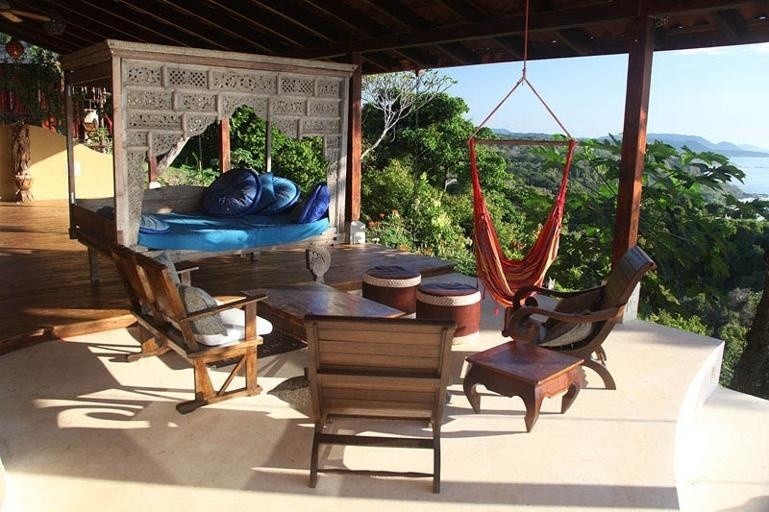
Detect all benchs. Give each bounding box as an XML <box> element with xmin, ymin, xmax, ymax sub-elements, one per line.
<box><xmin>104</xmin><ymin>237</ymin><xmax>267</xmax><ymax>413</ymax></box>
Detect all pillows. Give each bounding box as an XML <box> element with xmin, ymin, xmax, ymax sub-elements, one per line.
<box><xmin>200</xmin><ymin>167</ymin><xmax>327</xmax><ymax>224</ymax></box>
<box><xmin>151</xmin><ymin>252</ymin><xmax>225</xmax><ymax>337</ymax></box>
<box><xmin>542</xmin><ymin>288</ymin><xmax>600</xmax><ymax>346</ymax></box>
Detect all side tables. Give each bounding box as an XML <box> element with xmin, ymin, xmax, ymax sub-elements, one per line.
<box><xmin>462</xmin><ymin>340</ymin><xmax>587</xmax><ymax>430</ymax></box>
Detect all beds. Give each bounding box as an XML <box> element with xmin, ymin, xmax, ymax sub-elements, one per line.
<box><xmin>60</xmin><ymin>182</ymin><xmax>362</xmax><ymax>288</ymax></box>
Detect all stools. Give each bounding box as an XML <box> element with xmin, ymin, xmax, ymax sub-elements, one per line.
<box><xmin>363</xmin><ymin>265</ymin><xmax>483</xmax><ymax>335</ymax></box>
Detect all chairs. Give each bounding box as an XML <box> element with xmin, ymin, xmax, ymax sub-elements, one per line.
<box><xmin>502</xmin><ymin>247</ymin><xmax>654</xmax><ymax>389</ymax></box>
<box><xmin>305</xmin><ymin>315</ymin><xmax>456</xmax><ymax>494</ymax></box>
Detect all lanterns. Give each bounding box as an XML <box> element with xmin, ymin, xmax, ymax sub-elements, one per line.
<box><xmin>5</xmin><ymin>37</ymin><xmax>25</xmax><ymax>60</ymax></box>
